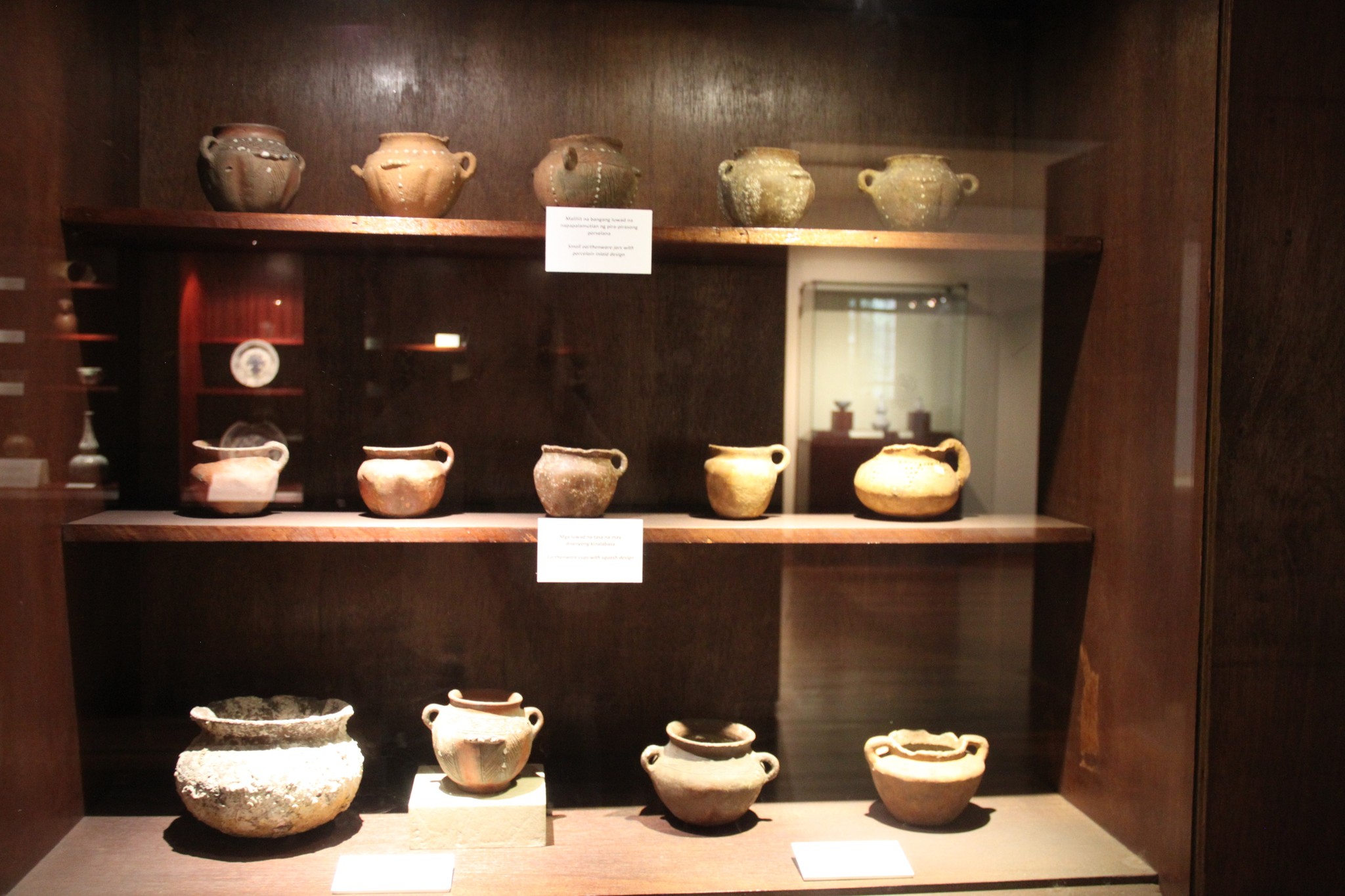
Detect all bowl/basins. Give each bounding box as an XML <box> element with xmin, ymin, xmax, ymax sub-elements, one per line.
<box><xmin>76</xmin><ymin>366</ymin><xmax>107</xmax><ymax>385</ymax></box>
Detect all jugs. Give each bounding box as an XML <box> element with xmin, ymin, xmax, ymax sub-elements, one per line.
<box><xmin>355</xmin><ymin>442</ymin><xmax>455</xmax><ymax>519</ymax></box>
<box><xmin>533</xmin><ymin>443</ymin><xmax>628</xmax><ymax>518</ymax></box>
<box><xmin>852</xmin><ymin>436</ymin><xmax>972</xmax><ymax>520</ymax></box>
<box><xmin>702</xmin><ymin>443</ymin><xmax>793</xmax><ymax>519</ymax></box>
<box><xmin>183</xmin><ymin>436</ymin><xmax>290</xmax><ymax>517</ymax></box>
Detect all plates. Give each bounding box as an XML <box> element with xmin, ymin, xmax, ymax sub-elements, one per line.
<box><xmin>231</xmin><ymin>338</ymin><xmax>280</xmax><ymax>388</ymax></box>
<box><xmin>217</xmin><ymin>420</ymin><xmax>288</xmax><ymax>461</ymax></box>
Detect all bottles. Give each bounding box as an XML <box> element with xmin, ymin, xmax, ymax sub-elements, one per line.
<box><xmin>640</xmin><ymin>717</ymin><xmax>780</xmax><ymax>828</ymax></box>
<box><xmin>350</xmin><ymin>132</ymin><xmax>478</xmax><ymax>218</ymax></box>
<box><xmin>717</xmin><ymin>145</ymin><xmax>816</xmax><ymax>228</ymax></box>
<box><xmin>421</xmin><ymin>686</ymin><xmax>544</xmax><ymax>794</ymax></box>
<box><xmin>863</xmin><ymin>728</ymin><xmax>990</xmax><ymax>827</ymax></box>
<box><xmin>173</xmin><ymin>694</ymin><xmax>366</xmax><ymax>839</ymax></box>
<box><xmin>65</xmin><ymin>410</ymin><xmax>110</xmax><ymax>484</ymax></box>
<box><xmin>53</xmin><ymin>297</ymin><xmax>77</xmax><ymax>334</ymax></box>
<box><xmin>858</xmin><ymin>152</ymin><xmax>981</xmax><ymax>232</ymax></box>
<box><xmin>531</xmin><ymin>133</ymin><xmax>642</xmax><ymax>211</ymax></box>
<box><xmin>196</xmin><ymin>122</ymin><xmax>306</xmax><ymax>213</ymax></box>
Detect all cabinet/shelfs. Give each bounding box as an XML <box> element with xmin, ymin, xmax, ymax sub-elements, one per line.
<box><xmin>0</xmin><ymin>204</ymin><xmax>1158</xmax><ymax>896</ymax></box>
